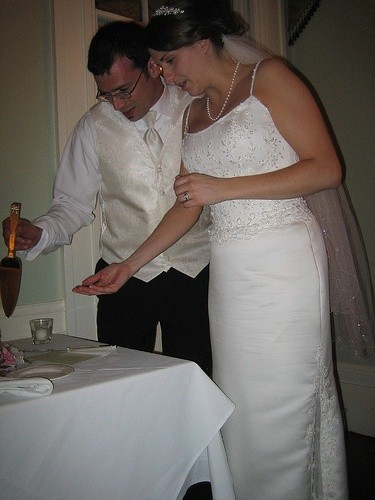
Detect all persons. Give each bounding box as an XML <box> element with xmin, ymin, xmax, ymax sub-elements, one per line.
<box><xmin>72</xmin><ymin>0</ymin><xmax>375</xmax><ymax>500</ymax></box>
<box><xmin>2</xmin><ymin>21</ymin><xmax>212</xmax><ymax>382</ymax></box>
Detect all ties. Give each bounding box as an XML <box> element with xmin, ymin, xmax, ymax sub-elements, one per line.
<box><xmin>142</xmin><ymin>109</ymin><xmax>164</xmax><ymax>162</ymax></box>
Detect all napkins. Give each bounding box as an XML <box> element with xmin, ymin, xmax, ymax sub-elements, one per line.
<box><xmin>0</xmin><ymin>377</ymin><xmax>54</xmax><ymax>396</ymax></box>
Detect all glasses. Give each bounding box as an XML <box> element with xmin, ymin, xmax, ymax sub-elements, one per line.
<box><xmin>95</xmin><ymin>69</ymin><xmax>146</xmax><ymax>102</ymax></box>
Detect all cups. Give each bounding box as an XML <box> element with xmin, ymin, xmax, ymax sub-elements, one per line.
<box><xmin>29</xmin><ymin>318</ymin><xmax>53</xmax><ymax>344</ymax></box>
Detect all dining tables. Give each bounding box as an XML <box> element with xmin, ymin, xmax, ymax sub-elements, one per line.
<box><xmin>0</xmin><ymin>334</ymin><xmax>235</xmax><ymax>500</ymax></box>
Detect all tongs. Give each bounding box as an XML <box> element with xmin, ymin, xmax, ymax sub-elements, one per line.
<box><xmin>67</xmin><ymin>343</ymin><xmax>117</xmax><ymax>353</ymax></box>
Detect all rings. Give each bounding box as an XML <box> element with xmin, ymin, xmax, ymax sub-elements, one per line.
<box><xmin>184</xmin><ymin>192</ymin><xmax>188</xmax><ymax>201</ymax></box>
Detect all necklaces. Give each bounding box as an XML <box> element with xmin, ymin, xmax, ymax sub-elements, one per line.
<box><xmin>207</xmin><ymin>62</ymin><xmax>241</xmax><ymax>120</ymax></box>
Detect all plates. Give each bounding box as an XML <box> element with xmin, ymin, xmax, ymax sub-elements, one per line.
<box><xmin>14</xmin><ymin>364</ymin><xmax>75</xmax><ymax>382</ymax></box>
